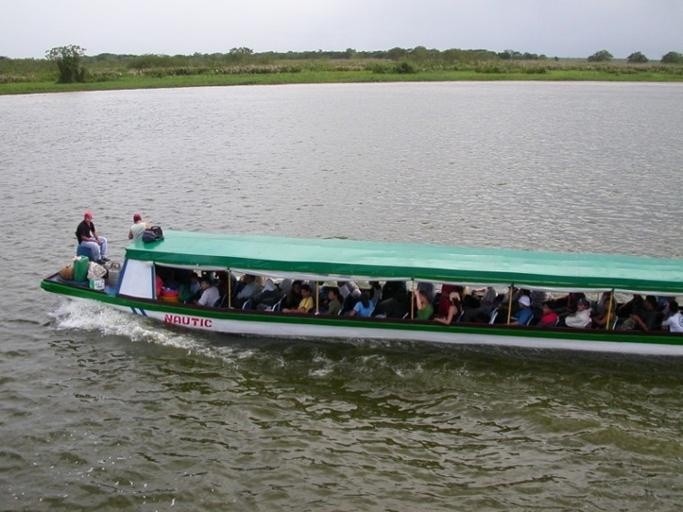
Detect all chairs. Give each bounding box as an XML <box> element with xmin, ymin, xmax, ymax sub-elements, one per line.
<box><xmin>213</xmin><ymin>286</ymin><xmax>619</xmax><ymax>331</ymax></box>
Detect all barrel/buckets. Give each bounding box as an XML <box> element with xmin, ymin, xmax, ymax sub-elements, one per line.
<box><xmin>74</xmin><ymin>255</ymin><xmax>88</xmax><ymax>281</ymax></box>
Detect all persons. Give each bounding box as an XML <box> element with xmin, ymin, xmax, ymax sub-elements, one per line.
<box><xmin>129</xmin><ymin>214</ymin><xmax>146</xmax><ymax>239</ymax></box>
<box><xmin>156</xmin><ymin>267</ymin><xmax>236</xmax><ymax>309</ymax></box>
<box><xmin>76</xmin><ymin>212</ymin><xmax>111</xmax><ymax>263</ymax></box>
<box><xmin>496</xmin><ymin>285</ymin><xmax>682</xmax><ymax>334</ymax></box>
<box><xmin>236</xmin><ymin>274</ymin><xmax>360</xmax><ymax>317</ymax></box>
<box><xmin>347</xmin><ymin>280</ymin><xmax>498</xmax><ymax>326</ymax></box>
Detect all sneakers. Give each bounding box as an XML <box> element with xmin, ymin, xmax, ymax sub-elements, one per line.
<box><xmin>97</xmin><ymin>259</ymin><xmax>105</xmax><ymax>264</ymax></box>
<box><xmin>101</xmin><ymin>257</ymin><xmax>110</xmax><ymax>261</ymax></box>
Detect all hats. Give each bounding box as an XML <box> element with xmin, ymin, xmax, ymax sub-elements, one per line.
<box><xmin>85</xmin><ymin>213</ymin><xmax>93</xmax><ymax>219</ymax></box>
<box><xmin>351</xmin><ymin>290</ymin><xmax>361</xmax><ymax>298</ymax></box>
<box><xmin>261</xmin><ymin>279</ymin><xmax>277</xmax><ymax>292</ymax></box>
<box><xmin>450</xmin><ymin>292</ymin><xmax>461</xmax><ymax>301</ymax></box>
<box><xmin>518</xmin><ymin>296</ymin><xmax>531</xmax><ymax>306</ymax></box>
<box><xmin>578</xmin><ymin>299</ymin><xmax>587</xmax><ymax>306</ymax></box>
<box><xmin>134</xmin><ymin>214</ymin><xmax>140</xmax><ymax>221</ymax></box>
<box><xmin>359</xmin><ymin>281</ymin><xmax>372</xmax><ymax>290</ymax></box>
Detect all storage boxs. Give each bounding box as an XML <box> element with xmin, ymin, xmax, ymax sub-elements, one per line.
<box><xmin>89</xmin><ymin>279</ymin><xmax>106</xmax><ymax>290</ymax></box>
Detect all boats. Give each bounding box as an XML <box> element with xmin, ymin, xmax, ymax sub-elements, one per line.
<box><xmin>39</xmin><ymin>230</ymin><xmax>683</xmax><ymax>357</ymax></box>
